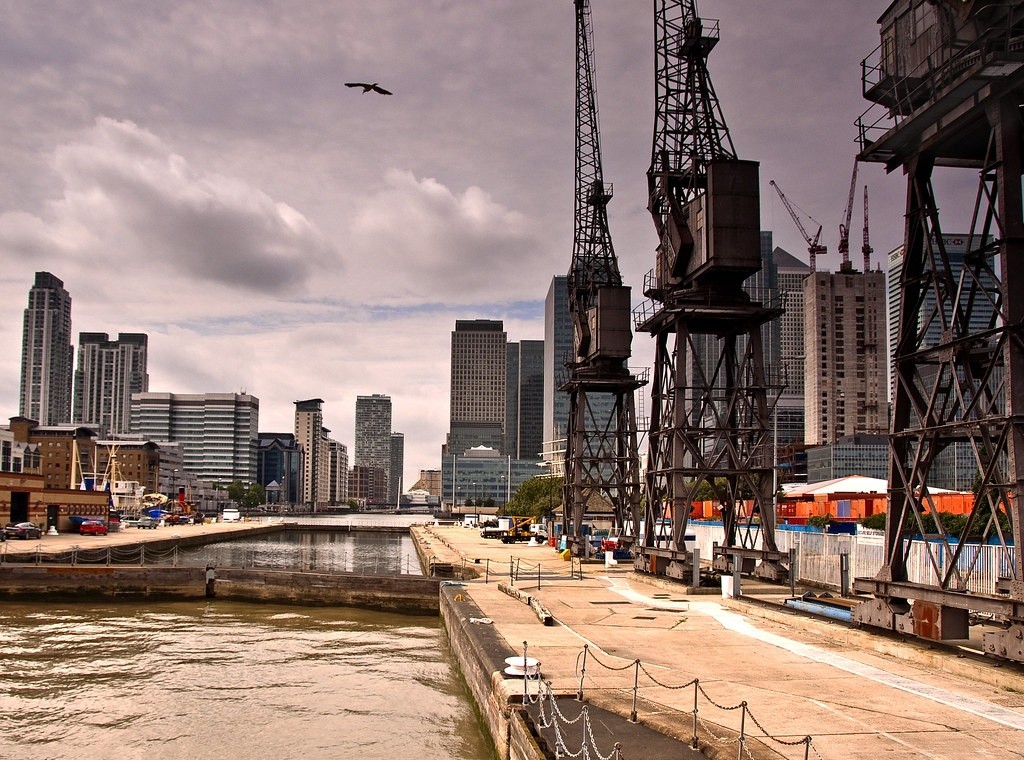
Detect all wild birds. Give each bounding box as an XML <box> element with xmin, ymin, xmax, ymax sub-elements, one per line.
<box><xmin>344</xmin><ymin>81</ymin><xmax>394</xmax><ymax>96</ymax></box>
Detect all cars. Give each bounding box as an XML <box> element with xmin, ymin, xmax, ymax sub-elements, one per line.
<box><xmin>137</xmin><ymin>517</ymin><xmax>157</xmax><ymax>530</ymax></box>
<box><xmin>4</xmin><ymin>522</ymin><xmax>43</xmax><ymax>540</ymax></box>
<box><xmin>121</xmin><ymin>516</ymin><xmax>139</xmax><ymax>527</ymax></box>
<box><xmin>80</xmin><ymin>520</ymin><xmax>108</xmax><ymax>536</ymax></box>
<box><xmin>0</xmin><ymin>523</ymin><xmax>7</xmax><ymax>542</ymax></box>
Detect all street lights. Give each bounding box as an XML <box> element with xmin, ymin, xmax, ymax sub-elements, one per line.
<box><xmin>247</xmin><ymin>481</ymin><xmax>252</xmax><ymax>517</ymax></box>
<box><xmin>473</xmin><ymin>483</ymin><xmax>477</xmax><ymax>528</ymax></box>
<box><xmin>216</xmin><ymin>478</ymin><xmax>222</xmax><ymax>517</ymax></box>
<box><xmin>457</xmin><ymin>487</ymin><xmax>461</xmax><ymax>522</ymax></box>
<box><xmin>173</xmin><ymin>468</ymin><xmax>178</xmax><ymax>516</ymax></box>
<box><xmin>501</xmin><ymin>475</ymin><xmax>506</xmax><ymax>516</ymax></box>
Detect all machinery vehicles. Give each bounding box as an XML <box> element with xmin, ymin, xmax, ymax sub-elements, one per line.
<box><xmin>497</xmin><ymin>513</ymin><xmax>549</xmax><ymax>545</ymax></box>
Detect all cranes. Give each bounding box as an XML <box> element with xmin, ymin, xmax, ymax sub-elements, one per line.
<box><xmin>549</xmin><ymin>0</ymin><xmax>799</xmax><ymax>598</ymax></box>
<box><xmin>769</xmin><ymin>178</ymin><xmax>830</xmax><ymax>273</ymax></box>
<box><xmin>835</xmin><ymin>154</ymin><xmax>875</xmax><ymax>274</ymax></box>
